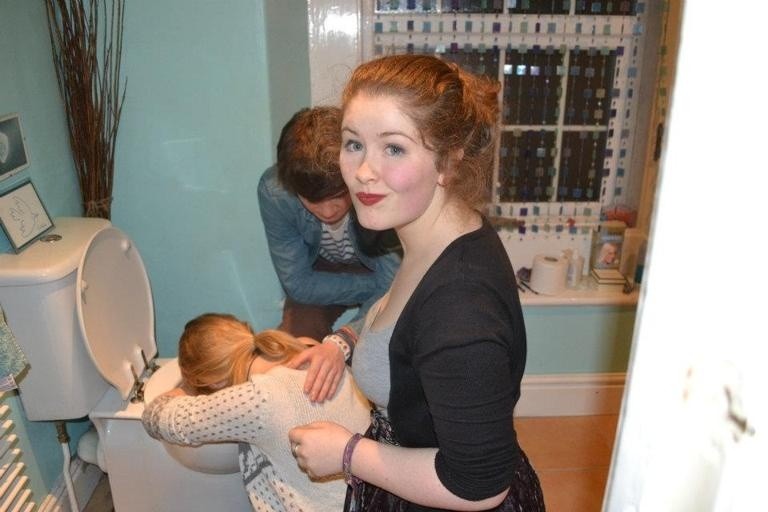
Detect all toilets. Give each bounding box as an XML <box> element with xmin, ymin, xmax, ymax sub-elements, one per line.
<box><xmin>0</xmin><ymin>218</ymin><xmax>253</xmax><ymax>512</ymax></box>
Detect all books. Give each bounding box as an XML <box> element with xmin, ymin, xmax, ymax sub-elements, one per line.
<box><xmin>590</xmin><ymin>268</ymin><xmax>627</xmax><ymax>285</ymax></box>
<box><xmin>589</xmin><ymin>220</ymin><xmax>627</xmax><ymax>270</ymax></box>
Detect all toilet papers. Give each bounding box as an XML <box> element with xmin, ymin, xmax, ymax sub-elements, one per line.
<box><xmin>530</xmin><ymin>254</ymin><xmax>567</xmax><ymax>296</ymax></box>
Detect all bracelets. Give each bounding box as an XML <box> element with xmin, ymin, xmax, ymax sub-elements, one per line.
<box><xmin>324</xmin><ymin>324</ymin><xmax>359</xmax><ymax>361</ymax></box>
<box><xmin>341</xmin><ymin>430</ymin><xmax>369</xmax><ymax>489</ymax></box>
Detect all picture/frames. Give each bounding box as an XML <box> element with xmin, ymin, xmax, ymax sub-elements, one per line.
<box><xmin>0</xmin><ymin>110</ymin><xmax>33</xmax><ymax>185</ymax></box>
<box><xmin>0</xmin><ymin>175</ymin><xmax>55</xmax><ymax>256</ymax></box>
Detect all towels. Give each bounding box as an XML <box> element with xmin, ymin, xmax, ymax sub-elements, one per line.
<box><xmin>1</xmin><ymin>305</ymin><xmax>29</xmax><ymax>402</ymax></box>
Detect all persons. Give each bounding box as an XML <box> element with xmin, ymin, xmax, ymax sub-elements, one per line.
<box><xmin>598</xmin><ymin>240</ymin><xmax>619</xmax><ymax>264</ymax></box>
<box><xmin>254</xmin><ymin>105</ymin><xmax>408</xmax><ymax>406</ymax></box>
<box><xmin>139</xmin><ymin>309</ymin><xmax>408</xmax><ymax>510</ymax></box>
<box><xmin>286</xmin><ymin>52</ymin><xmax>548</xmax><ymax>510</ymax></box>
